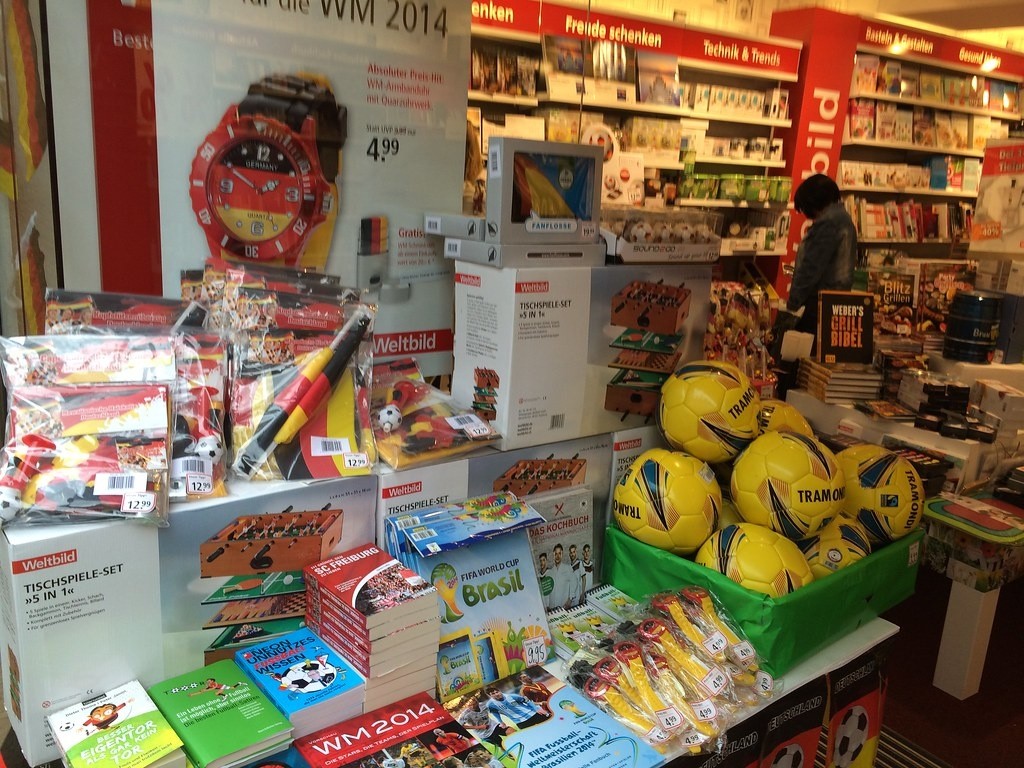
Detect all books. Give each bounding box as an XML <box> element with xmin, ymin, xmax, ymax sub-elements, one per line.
<box><xmin>46</xmin><ymin>483</ymin><xmax>667</xmax><ymax>768</ymax></box>
<box><xmin>795</xmin><ymin>51</ymin><xmax>1024</xmax><ymax>420</ymax></box>
<box><xmin>471</xmin><ymin>33</ymin><xmax>680</xmax><ymax>108</ymax></box>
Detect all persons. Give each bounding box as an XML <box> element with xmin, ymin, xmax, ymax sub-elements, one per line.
<box><xmin>568</xmin><ymin>544</ymin><xmax>587</xmax><ymax>593</ymax></box>
<box><xmin>398</xmin><ymin>741</ymin><xmax>426</xmax><ymax>768</ymax></box>
<box><xmin>580</xmin><ymin>544</ymin><xmax>593</xmax><ymax>591</ymax></box>
<box><xmin>517</xmin><ymin>671</ymin><xmax>552</xmax><ymax>705</ymax></box>
<box><xmin>483</xmin><ymin>685</ymin><xmax>546</xmax><ymax>730</ymax></box>
<box><xmin>377</xmin><ymin>756</ymin><xmax>411</xmax><ymax>768</ymax></box>
<box><xmin>786</xmin><ymin>173</ymin><xmax>858</xmax><ymax>357</ymax></box>
<box><xmin>441</xmin><ymin>756</ymin><xmax>466</xmax><ymax>768</ymax></box>
<box><xmin>475</xmin><ymin>748</ymin><xmax>506</xmax><ymax>768</ymax></box>
<box><xmin>547</xmin><ymin>545</ymin><xmax>580</xmax><ymax>608</ymax></box>
<box><xmin>464</xmin><ymin>752</ymin><xmax>482</xmax><ymax>768</ymax></box>
<box><xmin>457</xmin><ymin>696</ymin><xmax>517</xmax><ymax>749</ymax></box>
<box><xmin>536</xmin><ymin>553</ymin><xmax>553</xmax><ymax>612</ymax></box>
<box><xmin>432</xmin><ymin>728</ymin><xmax>472</xmax><ymax>755</ymax></box>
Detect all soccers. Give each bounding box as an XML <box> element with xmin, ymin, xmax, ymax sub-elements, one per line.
<box><xmin>612</xmin><ymin>358</ymin><xmax>925</xmax><ymax>606</ymax></box>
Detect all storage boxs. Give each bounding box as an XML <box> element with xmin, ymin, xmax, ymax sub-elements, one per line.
<box><xmin>0</xmin><ymin>73</ymin><xmax>791</xmax><ymax>767</ymax></box>
<box><xmin>599</xmin><ymin>521</ymin><xmax>927</xmax><ymax>680</ymax></box>
<box><xmin>838</xmin><ymin>51</ymin><xmax>1024</xmax><ymax>238</ymax></box>
<box><xmin>921</xmin><ymin>493</ymin><xmax>1024</xmax><ymax>594</ymax></box>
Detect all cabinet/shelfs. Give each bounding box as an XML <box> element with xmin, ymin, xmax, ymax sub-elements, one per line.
<box><xmin>463</xmin><ymin>23</ymin><xmax>804</xmax><ymax>256</ymax></box>
<box><xmin>835</xmin><ymin>45</ymin><xmax>1024</xmax><ymax>243</ymax></box>
